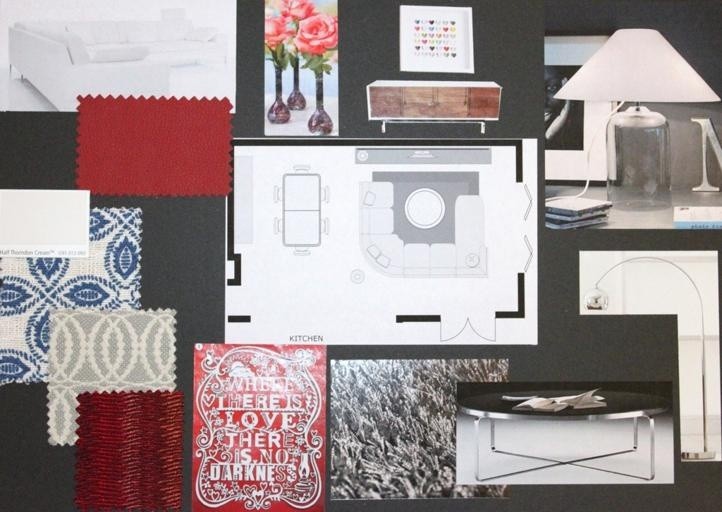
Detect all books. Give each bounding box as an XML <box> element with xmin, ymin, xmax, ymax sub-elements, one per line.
<box><xmin>672</xmin><ymin>206</ymin><xmax>722</xmax><ymax>231</ymax></box>
<box><xmin>544</xmin><ymin>197</ymin><xmax>614</xmax><ymax>231</ymax></box>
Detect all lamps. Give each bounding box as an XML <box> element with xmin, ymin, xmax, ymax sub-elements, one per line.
<box><xmin>552</xmin><ymin>27</ymin><xmax>722</xmax><ymax>213</ymax></box>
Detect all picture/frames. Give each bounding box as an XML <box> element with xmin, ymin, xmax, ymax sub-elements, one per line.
<box><xmin>543</xmin><ymin>31</ymin><xmax>624</xmax><ymax>188</ymax></box>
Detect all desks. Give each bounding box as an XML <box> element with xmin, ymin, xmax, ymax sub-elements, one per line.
<box><xmin>457</xmin><ymin>387</ymin><xmax>671</xmax><ymax>483</ymax></box>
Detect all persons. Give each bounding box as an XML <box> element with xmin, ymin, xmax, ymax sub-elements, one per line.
<box><xmin>543</xmin><ymin>66</ymin><xmax>572</xmax><ymax>150</ymax></box>
